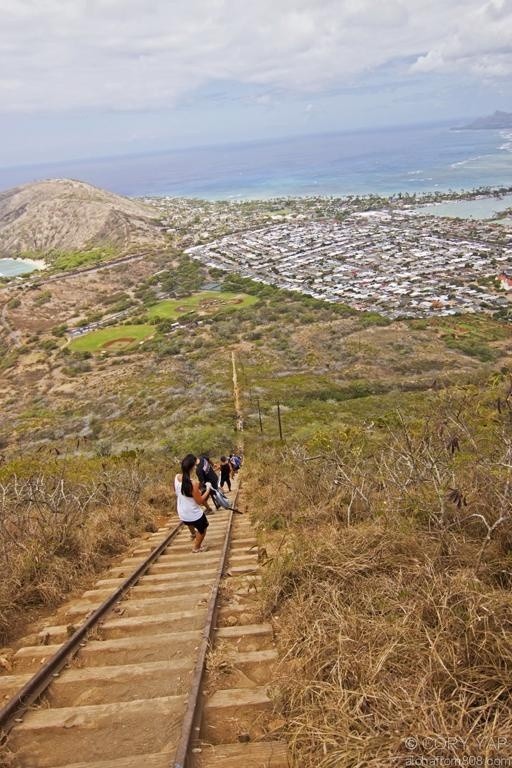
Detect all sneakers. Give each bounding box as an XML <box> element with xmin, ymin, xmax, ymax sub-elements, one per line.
<box><xmin>192</xmin><ymin>545</ymin><xmax>207</xmax><ymax>553</ymax></box>
<box><xmin>204</xmin><ymin>509</ymin><xmax>213</xmax><ymax>515</ymax></box>
<box><xmin>190</xmin><ymin>530</ymin><xmax>198</xmax><ymax>538</ymax></box>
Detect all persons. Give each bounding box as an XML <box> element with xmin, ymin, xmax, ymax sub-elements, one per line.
<box><xmin>175</xmin><ymin>451</ymin><xmax>241</xmax><ymax>553</ymax></box>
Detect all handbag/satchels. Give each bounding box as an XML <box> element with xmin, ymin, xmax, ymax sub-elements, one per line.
<box><xmin>215</xmin><ymin>491</ymin><xmax>230</xmax><ymax>508</ymax></box>
